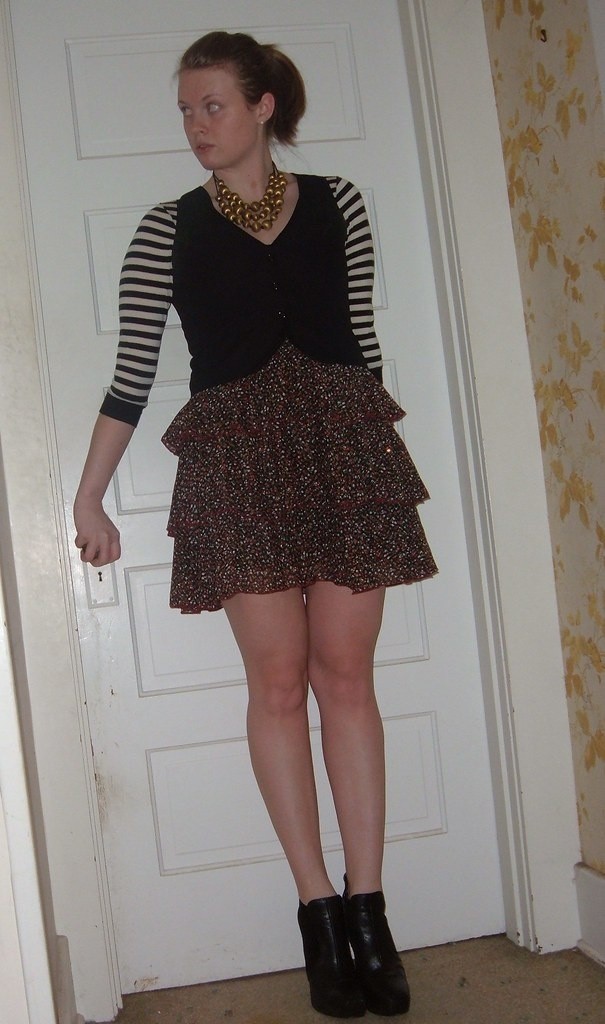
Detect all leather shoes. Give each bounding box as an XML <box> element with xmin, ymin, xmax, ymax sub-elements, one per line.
<box><xmin>298</xmin><ymin>895</ymin><xmax>367</xmax><ymax>1018</ymax></box>
<box><xmin>342</xmin><ymin>872</ymin><xmax>411</xmax><ymax>1016</ymax></box>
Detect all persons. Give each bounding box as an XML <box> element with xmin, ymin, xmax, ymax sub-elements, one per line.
<box><xmin>73</xmin><ymin>33</ymin><xmax>437</xmax><ymax>1020</ymax></box>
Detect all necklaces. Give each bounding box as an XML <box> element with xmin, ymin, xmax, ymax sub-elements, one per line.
<box><xmin>212</xmin><ymin>159</ymin><xmax>288</xmax><ymax>233</ymax></box>
